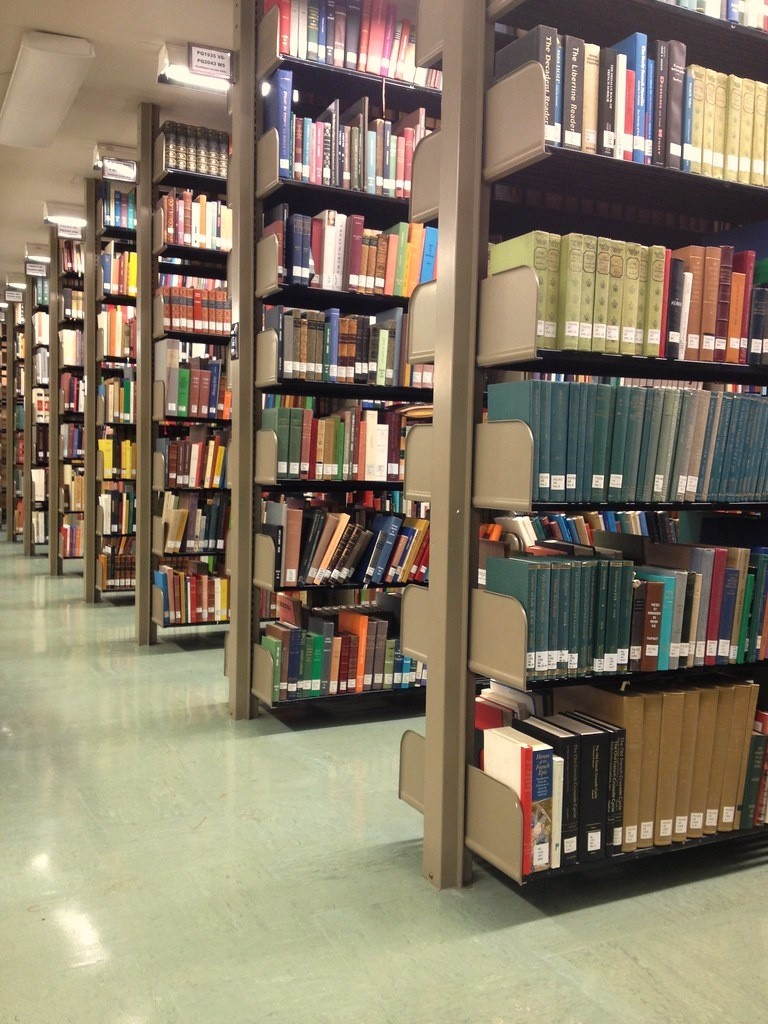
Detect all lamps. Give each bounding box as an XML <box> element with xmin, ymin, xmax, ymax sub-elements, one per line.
<box><xmin>0</xmin><ymin>295</ymin><xmax>9</xmax><ymax>308</ymax></box>
<box><xmin>92</xmin><ymin>144</ymin><xmax>135</xmax><ymax>169</ymax></box>
<box><xmin>5</xmin><ymin>272</ymin><xmax>27</xmax><ymax>289</ymax></box>
<box><xmin>24</xmin><ymin>243</ymin><xmax>51</xmax><ymax>262</ymax></box>
<box><xmin>156</xmin><ymin>43</ymin><xmax>230</xmax><ymax>94</ymax></box>
<box><xmin>41</xmin><ymin>202</ymin><xmax>88</xmax><ymax>226</ymax></box>
<box><xmin>0</xmin><ymin>30</ymin><xmax>97</xmax><ymax>151</ymax></box>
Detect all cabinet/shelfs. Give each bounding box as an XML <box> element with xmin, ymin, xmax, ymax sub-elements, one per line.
<box><xmin>0</xmin><ymin>304</ymin><xmax>10</xmax><ymax>533</ymax></box>
<box><xmin>49</xmin><ymin>225</ymin><xmax>88</xmax><ymax>575</ymax></box>
<box><xmin>5</xmin><ymin>287</ymin><xmax>25</xmax><ymax>544</ymax></box>
<box><xmin>229</xmin><ymin>0</ymin><xmax>427</xmax><ymax>728</ymax></box>
<box><xmin>135</xmin><ymin>100</ymin><xmax>233</xmax><ymax>646</ymax></box>
<box><xmin>21</xmin><ymin>259</ymin><xmax>51</xmax><ymax>561</ymax></box>
<box><xmin>400</xmin><ymin>0</ymin><xmax>765</xmax><ymax>896</ymax></box>
<box><xmin>81</xmin><ymin>174</ymin><xmax>140</xmax><ymax>604</ymax></box>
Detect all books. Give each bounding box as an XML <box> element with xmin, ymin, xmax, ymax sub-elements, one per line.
<box><xmin>0</xmin><ymin>1</ymin><xmax>768</xmax><ymax>884</ymax></box>
